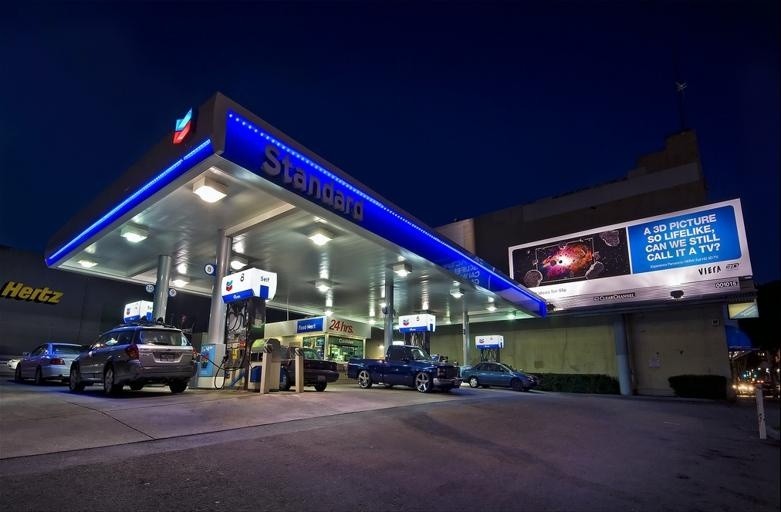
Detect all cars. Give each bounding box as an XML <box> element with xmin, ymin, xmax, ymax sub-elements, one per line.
<box><xmin>280</xmin><ymin>348</ymin><xmax>339</xmax><ymax>391</ymax></box>
<box><xmin>6</xmin><ymin>343</ymin><xmax>94</xmax><ymax>385</ymax></box>
<box><xmin>461</xmin><ymin>361</ymin><xmax>544</xmax><ymax>392</ymax></box>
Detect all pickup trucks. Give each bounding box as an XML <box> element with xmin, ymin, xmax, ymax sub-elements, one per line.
<box><xmin>347</xmin><ymin>345</ymin><xmax>463</xmax><ymax>392</ymax></box>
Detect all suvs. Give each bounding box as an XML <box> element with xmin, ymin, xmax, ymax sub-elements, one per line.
<box><xmin>69</xmin><ymin>319</ymin><xmax>197</xmax><ymax>393</ymax></box>
